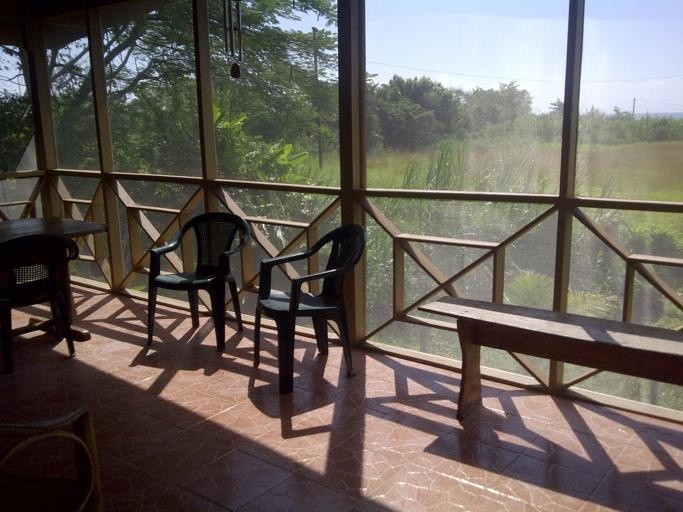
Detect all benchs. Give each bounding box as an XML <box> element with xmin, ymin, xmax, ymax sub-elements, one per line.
<box><xmin>417</xmin><ymin>296</ymin><xmax>683</xmax><ymax>420</ymax></box>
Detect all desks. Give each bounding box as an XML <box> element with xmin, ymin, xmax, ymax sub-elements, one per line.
<box><xmin>0</xmin><ymin>214</ymin><xmax>110</xmax><ymax>341</ymax></box>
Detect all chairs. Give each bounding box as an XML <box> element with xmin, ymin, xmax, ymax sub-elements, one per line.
<box><xmin>147</xmin><ymin>210</ymin><xmax>251</xmax><ymax>352</ymax></box>
<box><xmin>0</xmin><ymin>234</ymin><xmax>78</xmax><ymax>373</ymax></box>
<box><xmin>1</xmin><ymin>403</ymin><xmax>103</xmax><ymax>512</ymax></box>
<box><xmin>254</xmin><ymin>222</ymin><xmax>366</xmax><ymax>396</ymax></box>
